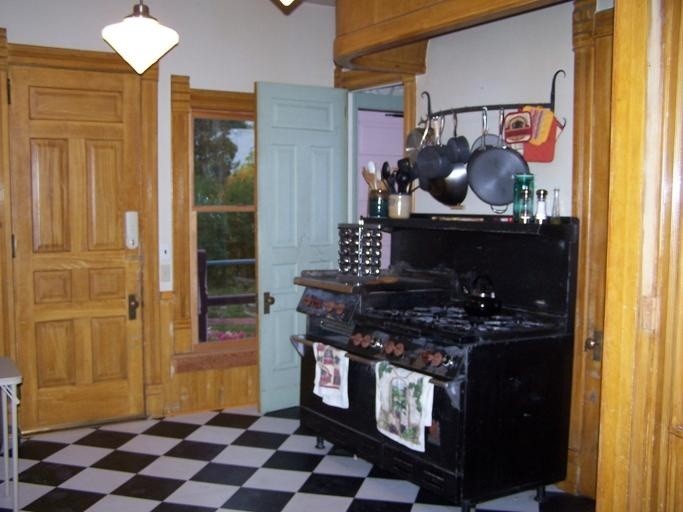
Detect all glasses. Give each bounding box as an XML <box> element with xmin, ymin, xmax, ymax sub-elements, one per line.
<box><xmin>101</xmin><ymin>0</ymin><xmax>180</xmax><ymax>75</ymax></box>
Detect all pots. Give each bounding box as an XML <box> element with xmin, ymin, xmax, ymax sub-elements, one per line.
<box><xmin>404</xmin><ymin>106</ymin><xmax>529</xmax><ymax>213</ymax></box>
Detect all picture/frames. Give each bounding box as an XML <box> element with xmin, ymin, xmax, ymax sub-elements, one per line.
<box><xmin>0</xmin><ymin>357</ymin><xmax>23</xmax><ymax>512</ymax></box>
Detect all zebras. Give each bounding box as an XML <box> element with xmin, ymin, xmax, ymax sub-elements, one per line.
<box><xmin>291</xmin><ymin>312</ymin><xmax>573</xmax><ymax>506</ymax></box>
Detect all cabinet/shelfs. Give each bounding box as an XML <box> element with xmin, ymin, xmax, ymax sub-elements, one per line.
<box><xmin>512</xmin><ymin>171</ymin><xmax>561</xmax><ymax>225</ymax></box>
<box><xmin>367</xmin><ymin>189</ymin><xmax>412</xmax><ymax>220</ymax></box>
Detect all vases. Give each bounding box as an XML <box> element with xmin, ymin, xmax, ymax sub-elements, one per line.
<box><xmin>206</xmin><ymin>330</ymin><xmax>247</xmax><ymax>341</ymax></box>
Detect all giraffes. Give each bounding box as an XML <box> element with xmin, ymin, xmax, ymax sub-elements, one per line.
<box><xmin>346</xmin><ymin>299</ymin><xmax>566</xmax><ymax>381</ymax></box>
